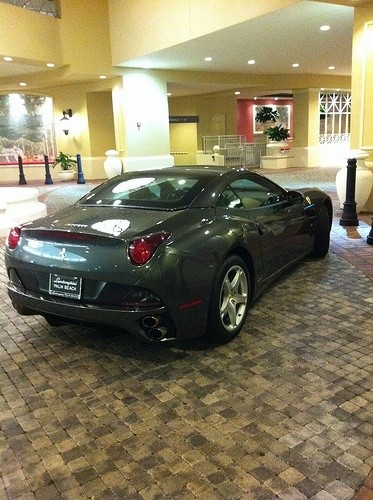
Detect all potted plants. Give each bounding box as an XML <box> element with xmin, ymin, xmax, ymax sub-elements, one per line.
<box><xmin>52</xmin><ymin>152</ymin><xmax>77</xmax><ymax>181</ymax></box>
<box><xmin>255</xmin><ymin>107</ymin><xmax>279</xmax><ymax>132</ymax></box>
<box><xmin>263</xmin><ymin>124</ymin><xmax>290</xmax><ymax>152</ymax></box>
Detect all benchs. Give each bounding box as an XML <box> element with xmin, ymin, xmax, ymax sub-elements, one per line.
<box><xmin>129</xmin><ymin>186</ymin><xmax>185</xmax><ymax>200</ymax></box>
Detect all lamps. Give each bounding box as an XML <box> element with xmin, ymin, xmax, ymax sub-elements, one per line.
<box><xmin>60</xmin><ymin>109</ymin><xmax>72</xmax><ymax>135</ymax></box>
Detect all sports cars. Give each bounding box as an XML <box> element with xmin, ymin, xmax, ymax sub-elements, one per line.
<box><xmin>3</xmin><ymin>164</ymin><xmax>334</xmax><ymax>347</ymax></box>
